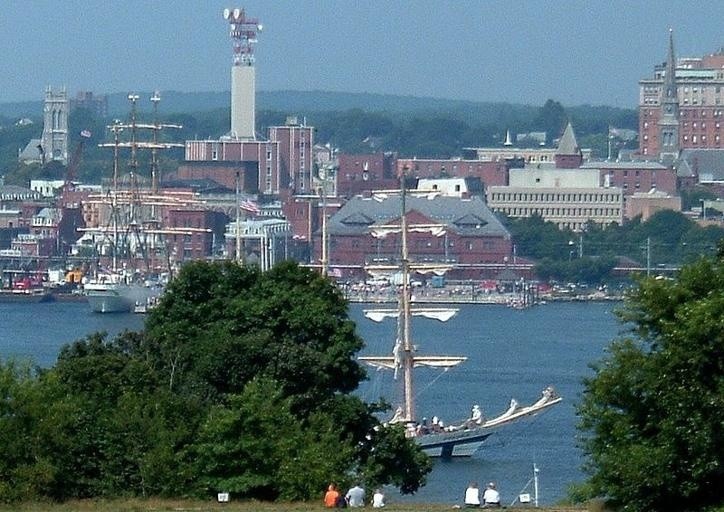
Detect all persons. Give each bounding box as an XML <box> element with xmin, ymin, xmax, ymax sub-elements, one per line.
<box><xmin>371</xmin><ymin>489</ymin><xmax>385</xmax><ymax>509</ymax></box>
<box><xmin>324</xmin><ymin>484</ymin><xmax>339</xmax><ymax>508</ymax></box>
<box><xmin>483</xmin><ymin>482</ymin><xmax>500</xmax><ymax>506</ymax></box>
<box><xmin>464</xmin><ymin>481</ymin><xmax>481</xmax><ymax>506</ymax></box>
<box><xmin>344</xmin><ymin>480</ymin><xmax>366</xmax><ymax>507</ymax></box>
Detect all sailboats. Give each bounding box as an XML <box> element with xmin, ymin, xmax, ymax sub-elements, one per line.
<box><xmin>336</xmin><ymin>167</ymin><xmax>564</xmax><ymax>459</ymax></box>
<box><xmin>82</xmin><ymin>92</ymin><xmax>211</xmax><ymax>314</ymax></box>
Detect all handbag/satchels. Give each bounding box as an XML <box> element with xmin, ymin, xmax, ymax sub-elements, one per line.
<box><xmin>336</xmin><ymin>497</ymin><xmax>346</xmax><ymax>508</ymax></box>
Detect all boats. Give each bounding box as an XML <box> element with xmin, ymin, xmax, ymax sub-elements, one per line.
<box><xmin>0</xmin><ymin>289</ymin><xmax>47</xmax><ymax>302</ymax></box>
<box><xmin>129</xmin><ymin>296</ymin><xmax>164</xmax><ymax>314</ymax></box>
